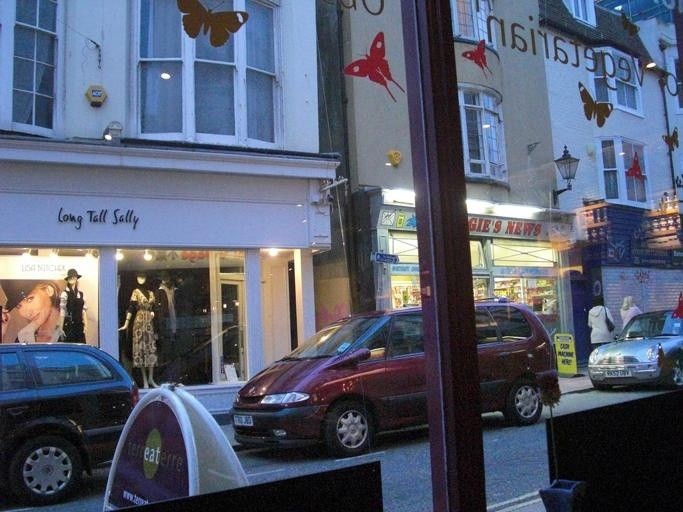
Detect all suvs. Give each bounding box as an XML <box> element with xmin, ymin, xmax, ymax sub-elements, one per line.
<box><xmin>0</xmin><ymin>338</ymin><xmax>144</xmax><ymax>509</ymax></box>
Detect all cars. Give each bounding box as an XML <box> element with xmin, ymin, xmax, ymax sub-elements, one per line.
<box><xmin>586</xmin><ymin>307</ymin><xmax>682</xmax><ymax>394</ymax></box>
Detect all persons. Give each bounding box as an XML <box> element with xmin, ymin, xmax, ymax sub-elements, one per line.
<box><xmin>0</xmin><ymin>280</ymin><xmax>59</xmax><ymax>344</ymax></box>
<box><xmin>154</xmin><ymin>270</ymin><xmax>187</xmax><ymax>387</ymax></box>
<box><xmin>118</xmin><ymin>272</ymin><xmax>161</xmax><ymax>389</ymax></box>
<box><xmin>54</xmin><ymin>268</ymin><xmax>87</xmax><ymax>344</ymax></box>
<box><xmin>587</xmin><ymin>296</ymin><xmax>613</xmax><ymax>347</ymax></box>
<box><xmin>618</xmin><ymin>295</ymin><xmax>642</xmax><ymax>330</ymax></box>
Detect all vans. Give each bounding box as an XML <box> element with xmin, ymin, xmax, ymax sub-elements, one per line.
<box><xmin>227</xmin><ymin>293</ymin><xmax>565</xmax><ymax>462</ymax></box>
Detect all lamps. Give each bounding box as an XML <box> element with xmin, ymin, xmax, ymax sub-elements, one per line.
<box><xmin>552</xmin><ymin>145</ymin><xmax>581</xmax><ymax>207</ymax></box>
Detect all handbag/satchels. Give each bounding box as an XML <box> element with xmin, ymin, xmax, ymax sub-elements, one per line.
<box><xmin>605</xmin><ymin>317</ymin><xmax>615</xmax><ymax>333</ymax></box>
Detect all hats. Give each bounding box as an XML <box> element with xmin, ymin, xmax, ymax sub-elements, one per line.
<box><xmin>64</xmin><ymin>269</ymin><xmax>82</xmax><ymax>281</ymax></box>
<box><xmin>0</xmin><ymin>280</ymin><xmax>43</xmax><ymax>312</ymax></box>
